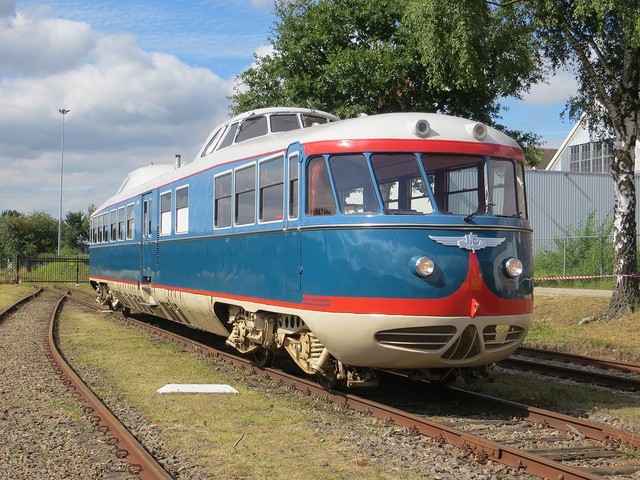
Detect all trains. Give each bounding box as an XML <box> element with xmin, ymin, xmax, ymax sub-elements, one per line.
<box><xmin>88</xmin><ymin>107</ymin><xmax>533</xmax><ymax>391</ymax></box>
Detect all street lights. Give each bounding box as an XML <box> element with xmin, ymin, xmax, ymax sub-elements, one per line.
<box><xmin>58</xmin><ymin>109</ymin><xmax>70</xmax><ymax>255</ymax></box>
